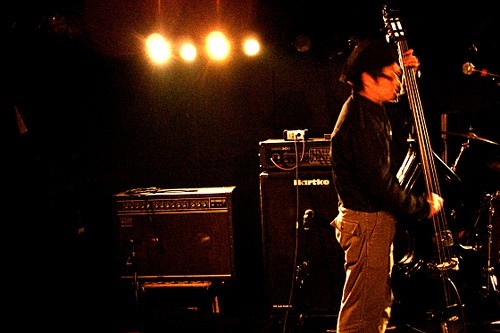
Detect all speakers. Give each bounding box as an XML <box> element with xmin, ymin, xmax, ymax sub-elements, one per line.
<box><xmin>113</xmin><ymin>186</ymin><xmax>239</xmax><ymax>281</ymax></box>
<box><xmin>260</xmin><ymin>170</ymin><xmax>346</xmax><ymax>321</ymax></box>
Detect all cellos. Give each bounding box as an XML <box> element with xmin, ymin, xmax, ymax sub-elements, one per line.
<box><xmin>379</xmin><ymin>0</ymin><xmax>497</xmax><ymax>333</ymax></box>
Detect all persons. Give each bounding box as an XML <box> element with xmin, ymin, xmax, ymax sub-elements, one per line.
<box><xmin>330</xmin><ymin>40</ymin><xmax>444</xmax><ymax>333</ymax></box>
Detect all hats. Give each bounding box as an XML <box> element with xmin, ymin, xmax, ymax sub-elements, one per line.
<box><xmin>339</xmin><ymin>35</ymin><xmax>397</xmax><ymax>87</ymax></box>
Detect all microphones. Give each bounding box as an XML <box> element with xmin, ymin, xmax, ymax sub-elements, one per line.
<box><xmin>303</xmin><ymin>209</ymin><xmax>315</xmax><ymax>227</ymax></box>
<box><xmin>462</xmin><ymin>62</ymin><xmax>500</xmax><ymax>81</ymax></box>
